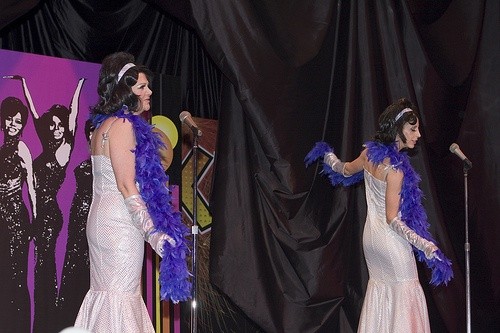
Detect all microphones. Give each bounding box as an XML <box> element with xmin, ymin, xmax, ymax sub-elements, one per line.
<box><xmin>179</xmin><ymin>111</ymin><xmax>202</xmax><ymax>136</ymax></box>
<box><xmin>449</xmin><ymin>143</ymin><xmax>472</xmax><ymax>168</ymax></box>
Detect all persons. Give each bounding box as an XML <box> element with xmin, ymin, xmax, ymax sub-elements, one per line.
<box><xmin>59</xmin><ymin>49</ymin><xmax>196</xmax><ymax>333</ymax></box>
<box><xmin>0</xmin><ymin>74</ymin><xmax>97</xmax><ymax>333</ymax></box>
<box><xmin>305</xmin><ymin>98</ymin><xmax>455</xmax><ymax>333</ymax></box>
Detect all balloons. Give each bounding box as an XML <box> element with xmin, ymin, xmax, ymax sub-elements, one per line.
<box><xmin>148</xmin><ymin>115</ymin><xmax>179</xmax><ymax>149</ymax></box>
<box><xmin>150</xmin><ymin>127</ymin><xmax>174</xmax><ymax>172</ymax></box>
